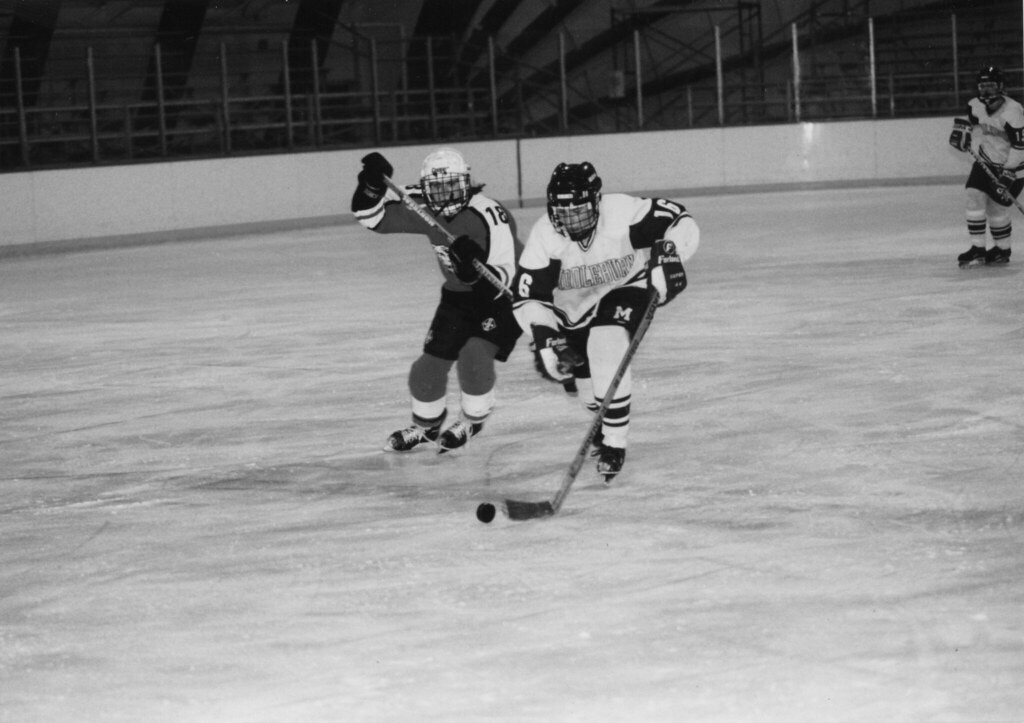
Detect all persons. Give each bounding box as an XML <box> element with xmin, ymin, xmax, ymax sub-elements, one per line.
<box><xmin>511</xmin><ymin>161</ymin><xmax>700</xmax><ymax>484</ymax></box>
<box><xmin>351</xmin><ymin>146</ymin><xmax>525</xmax><ymax>455</ymax></box>
<box><xmin>950</xmin><ymin>64</ymin><xmax>1024</xmax><ymax>270</ymax></box>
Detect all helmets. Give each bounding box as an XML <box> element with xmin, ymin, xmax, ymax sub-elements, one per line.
<box><xmin>547</xmin><ymin>162</ymin><xmax>602</xmax><ymax>239</ymax></box>
<box><xmin>421</xmin><ymin>149</ymin><xmax>469</xmax><ymax>216</ymax></box>
<box><xmin>975</xmin><ymin>64</ymin><xmax>1005</xmax><ymax>105</ymax></box>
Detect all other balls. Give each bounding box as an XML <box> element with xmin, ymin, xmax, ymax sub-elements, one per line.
<box><xmin>476</xmin><ymin>502</ymin><xmax>496</xmax><ymax>523</ymax></box>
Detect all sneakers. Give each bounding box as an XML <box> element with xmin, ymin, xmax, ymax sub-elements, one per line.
<box><xmin>383</xmin><ymin>407</ymin><xmax>447</xmax><ymax>452</ymax></box>
<box><xmin>438</xmin><ymin>409</ymin><xmax>491</xmax><ymax>454</ymax></box>
<box><xmin>957</xmin><ymin>244</ymin><xmax>988</xmax><ymax>270</ymax></box>
<box><xmin>591</xmin><ymin>427</ymin><xmax>603</xmax><ymax>447</ymax></box>
<box><xmin>984</xmin><ymin>246</ymin><xmax>1011</xmax><ymax>266</ymax></box>
<box><xmin>591</xmin><ymin>443</ymin><xmax>626</xmax><ymax>482</ymax></box>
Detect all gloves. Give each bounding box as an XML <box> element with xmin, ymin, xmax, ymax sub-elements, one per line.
<box><xmin>645</xmin><ymin>241</ymin><xmax>687</xmax><ymax>306</ymax></box>
<box><xmin>993</xmin><ymin>170</ymin><xmax>1016</xmax><ymax>201</ymax></box>
<box><xmin>449</xmin><ymin>235</ymin><xmax>486</xmax><ymax>284</ymax></box>
<box><xmin>357</xmin><ymin>153</ymin><xmax>393</xmax><ymax>199</ymax></box>
<box><xmin>530</xmin><ymin>323</ymin><xmax>585</xmax><ymax>381</ymax></box>
<box><xmin>948</xmin><ymin>118</ymin><xmax>972</xmax><ymax>151</ymax></box>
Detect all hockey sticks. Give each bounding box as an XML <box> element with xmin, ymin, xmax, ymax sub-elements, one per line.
<box><xmin>967</xmin><ymin>147</ymin><xmax>1024</xmax><ymax>215</ymax></box>
<box><xmin>502</xmin><ymin>290</ymin><xmax>660</xmax><ymax>522</ymax></box>
<box><xmin>383</xmin><ymin>173</ymin><xmax>513</xmax><ymax>303</ymax></box>
